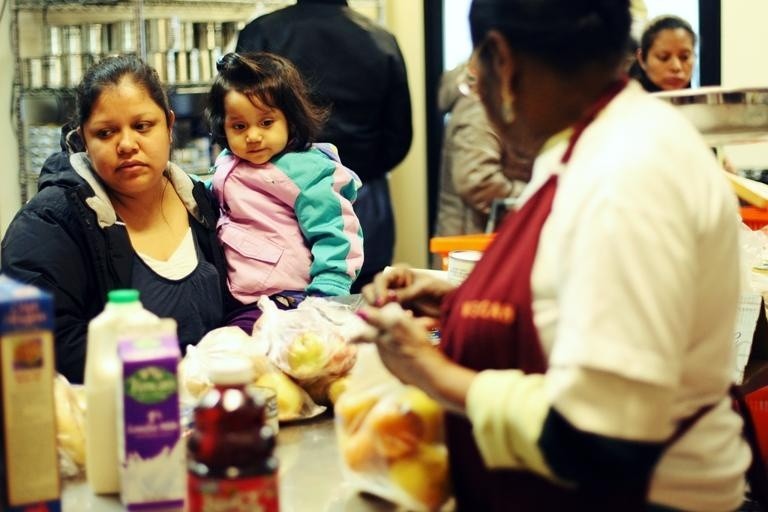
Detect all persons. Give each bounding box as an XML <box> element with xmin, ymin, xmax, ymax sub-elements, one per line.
<box><xmin>199</xmin><ymin>48</ymin><xmax>364</xmax><ymax>351</ymax></box>
<box><xmin>627</xmin><ymin>12</ymin><xmax>698</xmax><ymax>98</ymax></box>
<box><xmin>354</xmin><ymin>2</ymin><xmax>759</xmax><ymax>510</ymax></box>
<box><xmin>0</xmin><ymin>53</ymin><xmax>229</xmax><ymax>386</ymax></box>
<box><xmin>232</xmin><ymin>0</ymin><xmax>415</xmax><ymax>302</ymax></box>
<box><xmin>430</xmin><ymin>1</ymin><xmax>523</xmax><ymax>263</ymax></box>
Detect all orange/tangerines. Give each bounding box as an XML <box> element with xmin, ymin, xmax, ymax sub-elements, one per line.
<box><xmin>326</xmin><ymin>375</ymin><xmax>447</xmax><ymax>507</ymax></box>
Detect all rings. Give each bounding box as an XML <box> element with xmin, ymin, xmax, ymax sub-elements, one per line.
<box><xmin>379</xmin><ymin>262</ymin><xmax>397</xmax><ymax>287</ymax></box>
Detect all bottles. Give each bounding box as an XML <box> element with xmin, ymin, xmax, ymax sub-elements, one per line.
<box><xmin>81</xmin><ymin>286</ymin><xmax>178</xmax><ymax>498</ymax></box>
<box><xmin>186</xmin><ymin>358</ymin><xmax>284</xmax><ymax>510</ymax></box>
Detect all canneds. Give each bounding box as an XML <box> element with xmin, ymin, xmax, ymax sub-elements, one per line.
<box><xmin>20</xmin><ymin>17</ymin><xmax>244</xmax><ymax>92</ymax></box>
